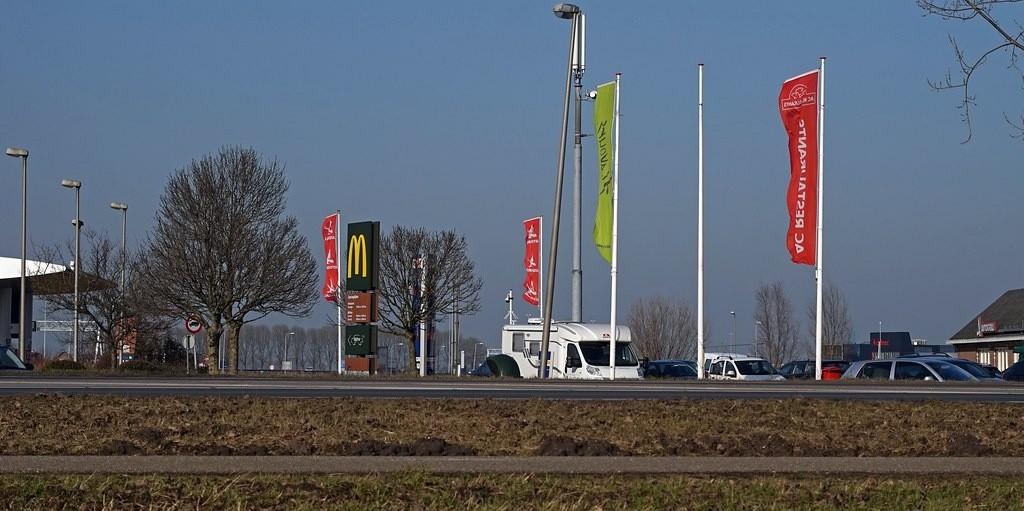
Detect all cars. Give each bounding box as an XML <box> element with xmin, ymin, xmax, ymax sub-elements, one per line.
<box><xmin>1001</xmin><ymin>361</ymin><xmax>1024</xmax><ymax>383</ymax></box>
<box><xmin>305</xmin><ymin>367</ymin><xmax>313</xmax><ymax>372</ymax></box>
<box><xmin>834</xmin><ymin>359</ymin><xmax>981</xmax><ymax>383</ymax></box>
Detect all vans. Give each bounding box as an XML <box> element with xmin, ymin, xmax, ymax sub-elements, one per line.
<box><xmin>644</xmin><ymin>359</ymin><xmax>708</xmax><ymax>379</ymax></box>
<box><xmin>704</xmin><ymin>353</ymin><xmax>750</xmax><ymax>378</ymax></box>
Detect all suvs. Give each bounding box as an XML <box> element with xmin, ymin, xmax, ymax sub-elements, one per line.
<box><xmin>897</xmin><ymin>351</ymin><xmax>1007</xmax><ymax>383</ymax></box>
<box><xmin>708</xmin><ymin>355</ymin><xmax>787</xmax><ymax>381</ymax></box>
<box><xmin>781</xmin><ymin>359</ymin><xmax>852</xmax><ymax>382</ymax></box>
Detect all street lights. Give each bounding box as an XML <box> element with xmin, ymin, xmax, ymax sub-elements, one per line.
<box><xmin>390</xmin><ymin>343</ymin><xmax>404</xmax><ymax>375</ymax></box>
<box><xmin>730</xmin><ymin>310</ymin><xmax>736</xmax><ymax>354</ymax></box>
<box><xmin>878</xmin><ymin>321</ymin><xmax>884</xmax><ymax>360</ymax></box>
<box><xmin>473</xmin><ymin>343</ymin><xmax>483</xmax><ymax>370</ymax></box>
<box><xmin>222</xmin><ymin>328</ymin><xmax>230</xmax><ymax>372</ymax></box>
<box><xmin>43</xmin><ymin>301</ymin><xmax>50</xmax><ymax>361</ymax></box>
<box><xmin>754</xmin><ymin>321</ymin><xmax>761</xmax><ymax>357</ymax></box>
<box><xmin>109</xmin><ymin>202</ymin><xmax>128</xmax><ymax>368</ymax></box>
<box><xmin>284</xmin><ymin>332</ymin><xmax>295</xmax><ymax>374</ymax></box>
<box><xmin>554</xmin><ymin>2</ymin><xmax>588</xmax><ymax>326</ymax></box>
<box><xmin>61</xmin><ymin>179</ymin><xmax>81</xmax><ymax>363</ymax></box>
<box><xmin>5</xmin><ymin>148</ymin><xmax>26</xmax><ymax>366</ymax></box>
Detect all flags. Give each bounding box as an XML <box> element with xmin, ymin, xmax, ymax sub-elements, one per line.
<box><xmin>779</xmin><ymin>72</ymin><xmax>818</xmax><ymax>265</ymax></box>
<box><xmin>594</xmin><ymin>83</ymin><xmax>616</xmax><ymax>264</ymax></box>
<box><xmin>322</xmin><ymin>215</ymin><xmax>338</xmax><ymax>301</ymax></box>
<box><xmin>523</xmin><ymin>219</ymin><xmax>540</xmax><ymax>306</ymax></box>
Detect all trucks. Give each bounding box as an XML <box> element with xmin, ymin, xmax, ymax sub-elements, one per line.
<box><xmin>501</xmin><ymin>322</ymin><xmax>645</xmax><ymax>380</ymax></box>
